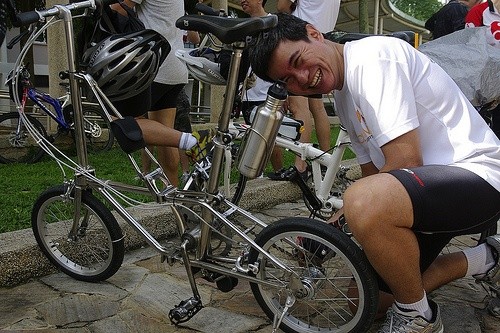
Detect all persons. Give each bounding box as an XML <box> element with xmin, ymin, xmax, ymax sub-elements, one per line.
<box><xmin>130</xmin><ymin>86</ymin><xmax>192</xmax><ymax>183</ymax></box>
<box><xmin>238</xmin><ymin>0</ymin><xmax>283</xmax><ymax>177</ymax></box>
<box><xmin>268</xmin><ymin>0</ymin><xmax>340</xmax><ymax>181</ymax></box>
<box><xmin>465</xmin><ymin>0</ymin><xmax>499</xmax><ymax>39</ymax></box>
<box><xmin>108</xmin><ymin>2</ymin><xmax>214</xmax><ymax>198</ymax></box>
<box><xmin>251</xmin><ymin>12</ymin><xmax>500</xmax><ymax>333</ymax></box>
<box><xmin>425</xmin><ymin>0</ymin><xmax>476</xmax><ymax>39</ymax></box>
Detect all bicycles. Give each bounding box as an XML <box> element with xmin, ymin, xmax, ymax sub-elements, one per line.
<box><xmin>0</xmin><ymin>67</ymin><xmax>117</xmax><ymax>163</ymax></box>
<box><xmin>173</xmin><ymin>2</ymin><xmax>425</xmax><ymax>257</ymax></box>
<box><xmin>3</xmin><ymin>0</ymin><xmax>379</xmax><ymax>333</ymax></box>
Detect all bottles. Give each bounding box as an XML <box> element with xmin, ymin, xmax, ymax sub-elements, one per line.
<box><xmin>247</xmin><ymin>106</ymin><xmax>304</xmax><ymax>142</ymax></box>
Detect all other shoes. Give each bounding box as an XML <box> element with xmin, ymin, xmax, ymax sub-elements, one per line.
<box><xmin>182</xmin><ymin>171</ymin><xmax>189</xmax><ymax>186</ymax></box>
<box><xmin>134</xmin><ymin>172</ymin><xmax>151</xmax><ymax>180</ymax></box>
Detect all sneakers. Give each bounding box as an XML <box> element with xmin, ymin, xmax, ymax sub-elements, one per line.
<box><xmin>366</xmin><ymin>300</ymin><xmax>444</xmax><ymax>333</ymax></box>
<box><xmin>471</xmin><ymin>234</ymin><xmax>500</xmax><ymax>318</ymax></box>
<box><xmin>268</xmin><ymin>165</ymin><xmax>308</xmax><ymax>183</ymax></box>
<box><xmin>185</xmin><ymin>128</ymin><xmax>215</xmax><ymax>183</ymax></box>
<box><xmin>307</xmin><ymin>165</ymin><xmax>327</xmax><ymax>175</ymax></box>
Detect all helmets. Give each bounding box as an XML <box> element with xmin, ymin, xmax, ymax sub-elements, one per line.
<box><xmin>175</xmin><ymin>46</ymin><xmax>227</xmax><ymax>86</ymax></box>
<box><xmin>82</xmin><ymin>27</ymin><xmax>171</xmax><ymax>104</ymax></box>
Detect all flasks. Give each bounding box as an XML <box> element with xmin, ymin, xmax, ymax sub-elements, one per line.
<box><xmin>237</xmin><ymin>84</ymin><xmax>288</xmax><ymax>179</ymax></box>
